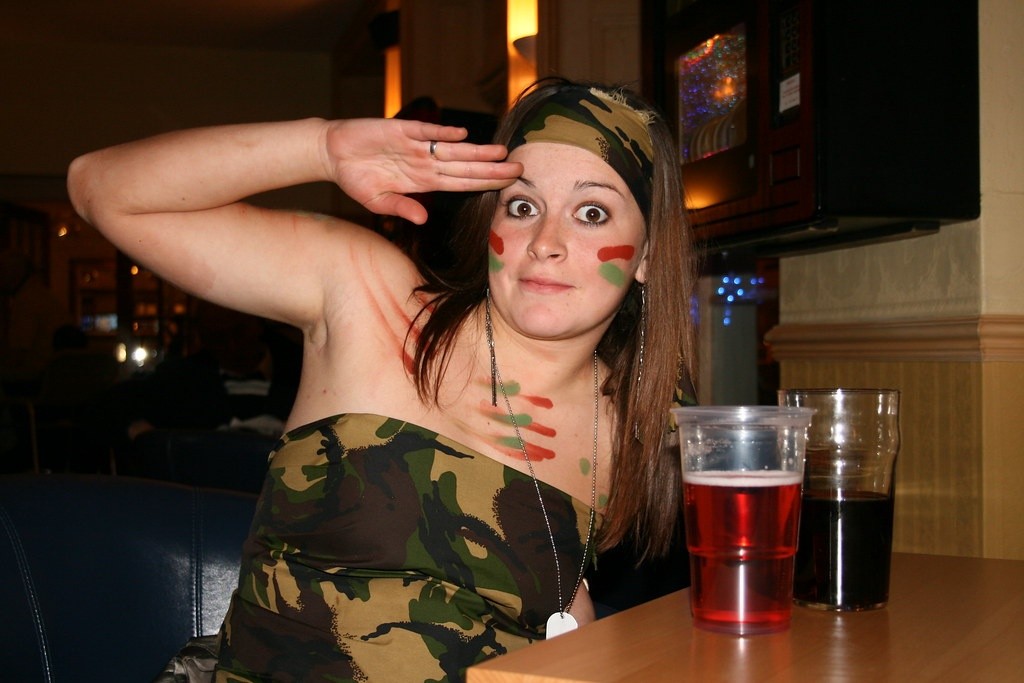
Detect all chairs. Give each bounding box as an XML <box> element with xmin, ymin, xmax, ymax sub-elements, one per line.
<box><xmin>0</xmin><ymin>415</ymin><xmax>277</xmax><ymax>683</ymax></box>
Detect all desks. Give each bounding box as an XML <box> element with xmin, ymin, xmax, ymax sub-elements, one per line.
<box><xmin>464</xmin><ymin>547</ymin><xmax>1024</xmax><ymax>683</ymax></box>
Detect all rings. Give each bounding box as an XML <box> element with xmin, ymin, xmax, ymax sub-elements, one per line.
<box><xmin>430</xmin><ymin>140</ymin><xmax>436</xmax><ymax>156</ymax></box>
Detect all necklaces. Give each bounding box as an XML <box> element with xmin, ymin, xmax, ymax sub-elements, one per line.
<box><xmin>486</xmin><ymin>291</ymin><xmax>599</xmax><ymax>639</ymax></box>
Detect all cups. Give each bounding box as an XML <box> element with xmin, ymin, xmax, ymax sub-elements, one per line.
<box><xmin>670</xmin><ymin>406</ymin><xmax>817</xmax><ymax>634</ymax></box>
<box><xmin>777</xmin><ymin>387</ymin><xmax>900</xmax><ymax>612</ymax></box>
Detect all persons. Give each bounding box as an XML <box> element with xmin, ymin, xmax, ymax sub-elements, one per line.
<box><xmin>67</xmin><ymin>70</ymin><xmax>700</xmax><ymax>683</ymax></box>
<box><xmin>39</xmin><ymin>326</ymin><xmax>121</xmax><ymax>427</ymax></box>
